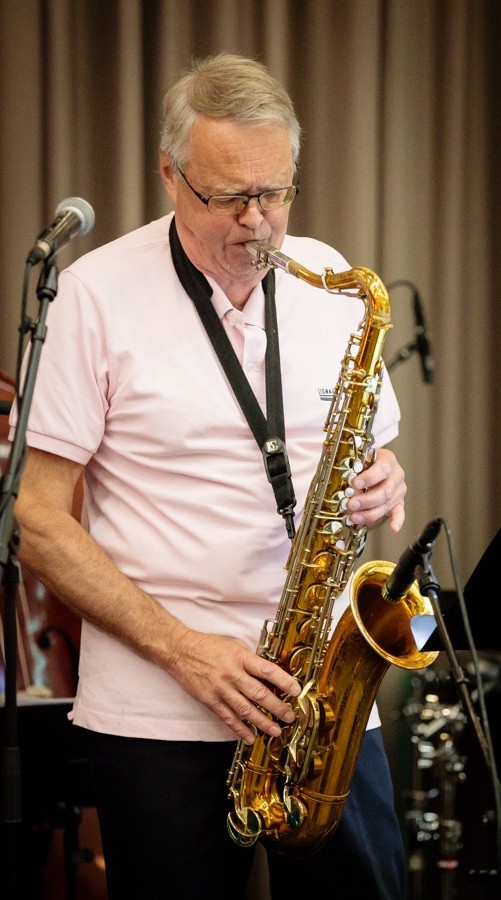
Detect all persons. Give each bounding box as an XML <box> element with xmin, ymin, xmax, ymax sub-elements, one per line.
<box><xmin>12</xmin><ymin>56</ymin><xmax>407</xmax><ymax>900</ymax></box>
<box><xmin>0</xmin><ymin>377</ymin><xmax>24</xmax><ymax>697</ymax></box>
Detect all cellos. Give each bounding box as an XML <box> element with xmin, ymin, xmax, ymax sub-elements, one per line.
<box><xmin>0</xmin><ymin>373</ymin><xmax>108</xmax><ymax>900</ymax></box>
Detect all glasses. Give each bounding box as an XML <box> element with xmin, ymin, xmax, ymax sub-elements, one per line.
<box><xmin>168</xmin><ymin>148</ymin><xmax>300</xmax><ymax>216</ymax></box>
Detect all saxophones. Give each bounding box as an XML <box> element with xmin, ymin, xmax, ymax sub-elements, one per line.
<box><xmin>223</xmin><ymin>236</ymin><xmax>439</xmax><ymax>853</ymax></box>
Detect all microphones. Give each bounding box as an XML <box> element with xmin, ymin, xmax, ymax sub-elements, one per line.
<box><xmin>380</xmin><ymin>516</ymin><xmax>445</xmax><ymax>606</ymax></box>
<box><xmin>412</xmin><ymin>290</ymin><xmax>436</xmax><ymax>386</ymax></box>
<box><xmin>26</xmin><ymin>194</ymin><xmax>96</xmax><ymax>266</ymax></box>
